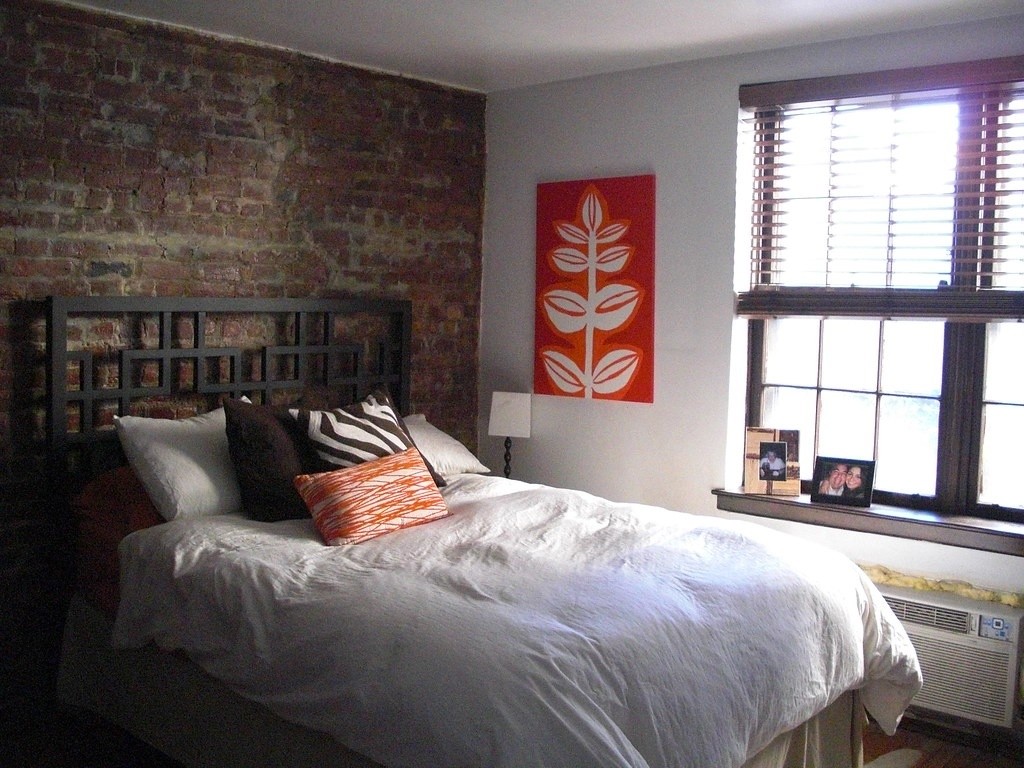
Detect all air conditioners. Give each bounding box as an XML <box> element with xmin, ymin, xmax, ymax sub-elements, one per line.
<box><xmin>873</xmin><ymin>582</ymin><xmax>1023</xmax><ymax>730</ymax></box>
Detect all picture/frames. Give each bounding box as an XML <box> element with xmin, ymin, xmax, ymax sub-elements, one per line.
<box><xmin>811</xmin><ymin>455</ymin><xmax>873</xmax><ymax>507</ymax></box>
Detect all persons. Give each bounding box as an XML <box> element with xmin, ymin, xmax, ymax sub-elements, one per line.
<box><xmin>818</xmin><ymin>462</ymin><xmax>868</xmax><ymax>498</ymax></box>
<box><xmin>760</xmin><ymin>448</ymin><xmax>786</xmax><ymax>480</ymax></box>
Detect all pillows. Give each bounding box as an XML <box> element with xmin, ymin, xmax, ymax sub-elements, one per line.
<box><xmin>401</xmin><ymin>412</ymin><xmax>493</xmax><ymax>479</ymax></box>
<box><xmin>112</xmin><ymin>395</ymin><xmax>254</xmax><ymax>521</ymax></box>
<box><xmin>220</xmin><ymin>384</ymin><xmax>331</xmax><ymax>522</ymax></box>
<box><xmin>291</xmin><ymin>445</ymin><xmax>455</xmax><ymax>547</ymax></box>
<box><xmin>278</xmin><ymin>383</ymin><xmax>448</xmax><ymax>490</ymax></box>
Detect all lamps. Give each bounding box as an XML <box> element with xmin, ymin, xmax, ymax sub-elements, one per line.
<box><xmin>488</xmin><ymin>391</ymin><xmax>531</xmax><ymax>476</ymax></box>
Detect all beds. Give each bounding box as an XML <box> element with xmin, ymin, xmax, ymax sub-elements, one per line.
<box><xmin>49</xmin><ymin>295</ymin><xmax>921</xmax><ymax>768</ymax></box>
